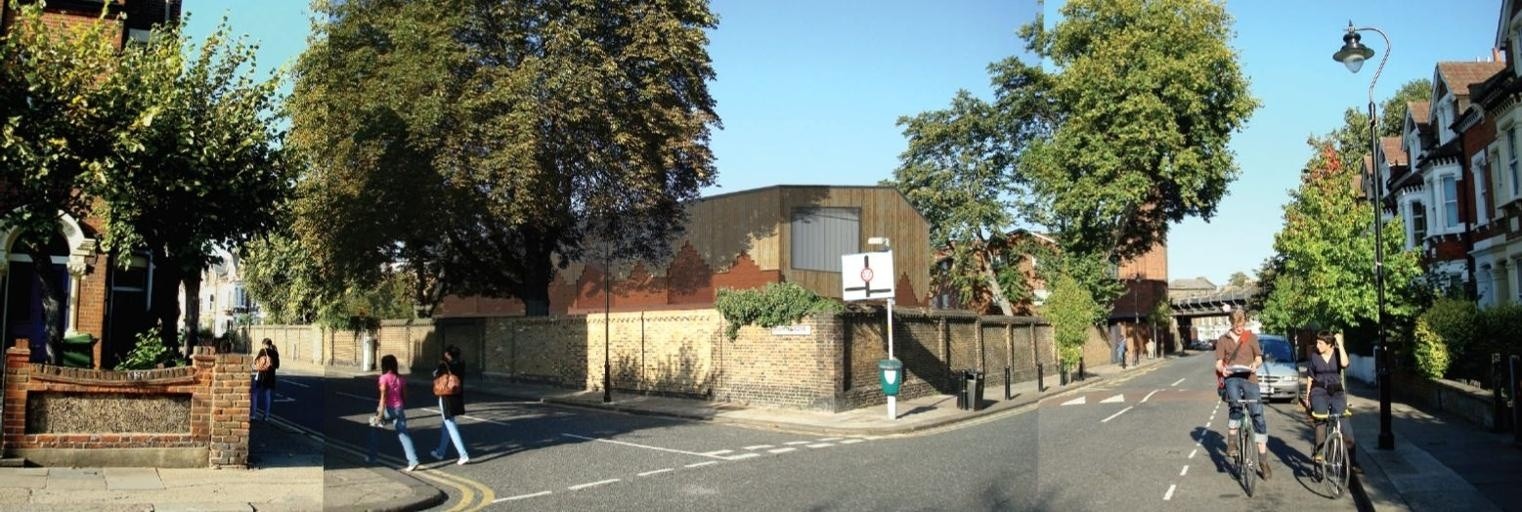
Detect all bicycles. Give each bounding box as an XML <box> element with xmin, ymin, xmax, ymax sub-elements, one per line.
<box><xmin>1305</xmin><ymin>398</ymin><xmax>1353</xmax><ymax>499</ymax></box>
<box><xmin>1223</xmin><ymin>385</ymin><xmax>1269</xmax><ymax>498</ymax></box>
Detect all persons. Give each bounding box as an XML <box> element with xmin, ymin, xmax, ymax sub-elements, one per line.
<box><xmin>1214</xmin><ymin>304</ymin><xmax>1273</xmax><ymax>482</ymax></box>
<box><xmin>248</xmin><ymin>336</ymin><xmax>280</xmax><ymax>423</ymax></box>
<box><xmin>363</xmin><ymin>355</ymin><xmax>420</xmax><ymax>472</ymax></box>
<box><xmin>1144</xmin><ymin>337</ymin><xmax>1156</xmax><ymax>359</ymax></box>
<box><xmin>1115</xmin><ymin>335</ymin><xmax>1127</xmax><ymax>366</ymax></box>
<box><xmin>429</xmin><ymin>344</ymin><xmax>470</xmax><ymax>466</ymax></box>
<box><xmin>1304</xmin><ymin>330</ymin><xmax>1364</xmax><ymax>474</ymax></box>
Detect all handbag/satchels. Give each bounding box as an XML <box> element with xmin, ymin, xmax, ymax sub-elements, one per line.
<box><xmin>1217</xmin><ymin>365</ymin><xmax>1228</xmax><ymax>401</ymax></box>
<box><xmin>254</xmin><ymin>348</ymin><xmax>272</xmax><ymax>371</ymax></box>
<box><xmin>432</xmin><ymin>361</ymin><xmax>460</xmax><ymax>396</ymax></box>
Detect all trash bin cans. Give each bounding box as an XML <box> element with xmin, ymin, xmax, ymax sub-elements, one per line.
<box><xmin>62</xmin><ymin>332</ymin><xmax>100</xmax><ymax>369</ymax></box>
<box><xmin>958</xmin><ymin>370</ymin><xmax>984</xmax><ymax>411</ymax></box>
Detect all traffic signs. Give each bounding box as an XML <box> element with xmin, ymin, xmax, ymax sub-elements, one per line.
<box><xmin>841</xmin><ymin>250</ymin><xmax>896</xmax><ymax>301</ymax></box>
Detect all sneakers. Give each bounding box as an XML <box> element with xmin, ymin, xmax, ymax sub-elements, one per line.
<box><xmin>1258</xmin><ymin>459</ymin><xmax>1272</xmax><ymax>480</ymax></box>
<box><xmin>1226</xmin><ymin>443</ymin><xmax>1241</xmax><ymax>457</ymax></box>
<box><xmin>405</xmin><ymin>462</ymin><xmax>419</xmax><ymax>473</ymax></box>
<box><xmin>430</xmin><ymin>451</ymin><xmax>444</xmax><ymax>460</ymax></box>
<box><xmin>457</xmin><ymin>456</ymin><xmax>470</xmax><ymax>466</ymax></box>
<box><xmin>1351</xmin><ymin>463</ymin><xmax>1365</xmax><ymax>476</ymax></box>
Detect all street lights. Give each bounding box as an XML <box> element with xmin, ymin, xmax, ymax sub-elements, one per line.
<box><xmin>1135</xmin><ymin>272</ymin><xmax>1141</xmax><ymax>364</ymax></box>
<box><xmin>1331</xmin><ymin>18</ymin><xmax>1396</xmax><ymax>453</ymax></box>
<box><xmin>867</xmin><ymin>236</ymin><xmax>896</xmax><ymax>421</ymax></box>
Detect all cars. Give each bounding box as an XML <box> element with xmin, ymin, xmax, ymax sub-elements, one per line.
<box><xmin>1255</xmin><ymin>335</ymin><xmax>1301</xmax><ymax>405</ymax></box>
